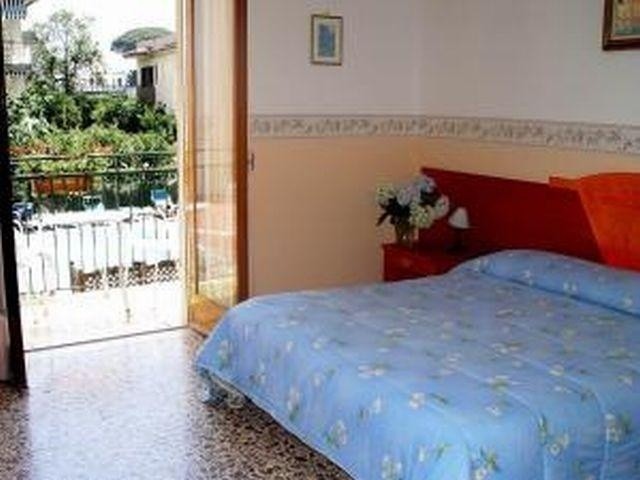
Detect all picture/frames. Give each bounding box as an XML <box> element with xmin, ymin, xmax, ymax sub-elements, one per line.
<box><xmin>310</xmin><ymin>14</ymin><xmax>343</xmax><ymax>65</ymax></box>
<box><xmin>602</xmin><ymin>0</ymin><xmax>639</xmax><ymax>49</ymax></box>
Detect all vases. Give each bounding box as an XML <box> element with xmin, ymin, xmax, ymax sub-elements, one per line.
<box><xmin>395</xmin><ymin>217</ymin><xmax>419</xmax><ymax>244</ymax></box>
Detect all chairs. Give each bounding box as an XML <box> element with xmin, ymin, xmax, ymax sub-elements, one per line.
<box><xmin>149</xmin><ymin>188</ymin><xmax>181</xmax><ymax>221</ymax></box>
<box><xmin>11</xmin><ymin>201</ymin><xmax>40</xmax><ymax>234</ymax></box>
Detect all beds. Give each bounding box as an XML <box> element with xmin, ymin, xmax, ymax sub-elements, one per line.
<box><xmin>191</xmin><ymin>173</ymin><xmax>640</xmax><ymax>480</ymax></box>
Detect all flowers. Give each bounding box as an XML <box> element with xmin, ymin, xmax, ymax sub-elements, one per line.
<box><xmin>372</xmin><ymin>175</ymin><xmax>469</xmax><ymax>239</ymax></box>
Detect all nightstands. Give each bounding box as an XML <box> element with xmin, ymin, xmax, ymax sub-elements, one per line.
<box><xmin>382</xmin><ymin>243</ymin><xmax>478</xmax><ymax>282</ymax></box>
